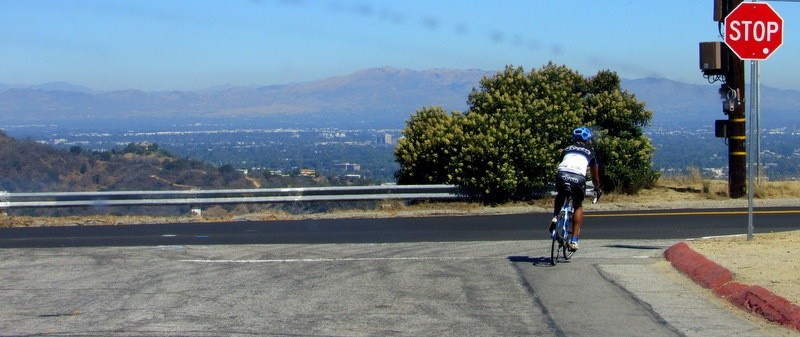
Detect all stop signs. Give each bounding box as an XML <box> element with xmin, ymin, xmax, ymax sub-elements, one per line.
<box><xmin>724</xmin><ymin>1</ymin><xmax>784</xmax><ymax>60</ymax></box>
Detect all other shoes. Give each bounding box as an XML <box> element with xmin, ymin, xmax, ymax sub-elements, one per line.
<box><xmin>549</xmin><ymin>216</ymin><xmax>557</xmax><ymax>232</ymax></box>
<box><xmin>570</xmin><ymin>242</ymin><xmax>579</xmax><ymax>250</ymax></box>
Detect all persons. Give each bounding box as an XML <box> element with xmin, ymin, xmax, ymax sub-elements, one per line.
<box><xmin>550</xmin><ymin>127</ymin><xmax>602</xmax><ymax>252</ymax></box>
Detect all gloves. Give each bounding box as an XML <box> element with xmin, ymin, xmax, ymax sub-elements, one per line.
<box><xmin>593</xmin><ymin>185</ymin><xmax>602</xmax><ymax>200</ymax></box>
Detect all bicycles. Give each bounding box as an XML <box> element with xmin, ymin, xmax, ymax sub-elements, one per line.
<box><xmin>550</xmin><ymin>188</ymin><xmax>602</xmax><ymax>266</ymax></box>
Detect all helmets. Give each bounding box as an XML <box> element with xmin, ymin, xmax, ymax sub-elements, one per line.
<box><xmin>573</xmin><ymin>127</ymin><xmax>593</xmax><ymax>143</ymax></box>
